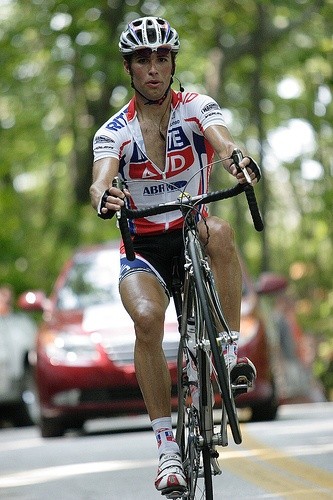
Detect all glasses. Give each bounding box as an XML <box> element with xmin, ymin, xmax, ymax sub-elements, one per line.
<box><xmin>129</xmin><ymin>44</ymin><xmax>177</xmax><ymax>56</ymax></box>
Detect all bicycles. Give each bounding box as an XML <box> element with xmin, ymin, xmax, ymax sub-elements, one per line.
<box><xmin>110</xmin><ymin>149</ymin><xmax>265</xmax><ymax>500</ymax></box>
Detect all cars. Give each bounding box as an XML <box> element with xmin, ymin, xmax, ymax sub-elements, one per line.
<box><xmin>16</xmin><ymin>240</ymin><xmax>288</xmax><ymax>438</ymax></box>
<box><xmin>0</xmin><ymin>283</ymin><xmax>41</xmax><ymax>428</ymax></box>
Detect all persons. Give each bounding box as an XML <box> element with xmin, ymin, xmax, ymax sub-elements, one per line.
<box><xmin>88</xmin><ymin>18</ymin><xmax>262</xmax><ymax>491</ymax></box>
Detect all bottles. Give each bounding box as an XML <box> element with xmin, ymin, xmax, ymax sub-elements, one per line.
<box><xmin>186</xmin><ymin>317</ymin><xmax>197</xmax><ymax>361</ymax></box>
<box><xmin>187</xmin><ymin>359</ymin><xmax>215</xmax><ymax>411</ymax></box>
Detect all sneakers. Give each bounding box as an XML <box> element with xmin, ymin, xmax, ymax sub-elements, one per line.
<box><xmin>221</xmin><ymin>344</ymin><xmax>256</xmax><ymax>396</ymax></box>
<box><xmin>155</xmin><ymin>430</ymin><xmax>187</xmax><ymax>492</ymax></box>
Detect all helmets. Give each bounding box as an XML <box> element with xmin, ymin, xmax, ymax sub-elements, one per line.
<box><xmin>119</xmin><ymin>16</ymin><xmax>180</xmax><ymax>56</ymax></box>
